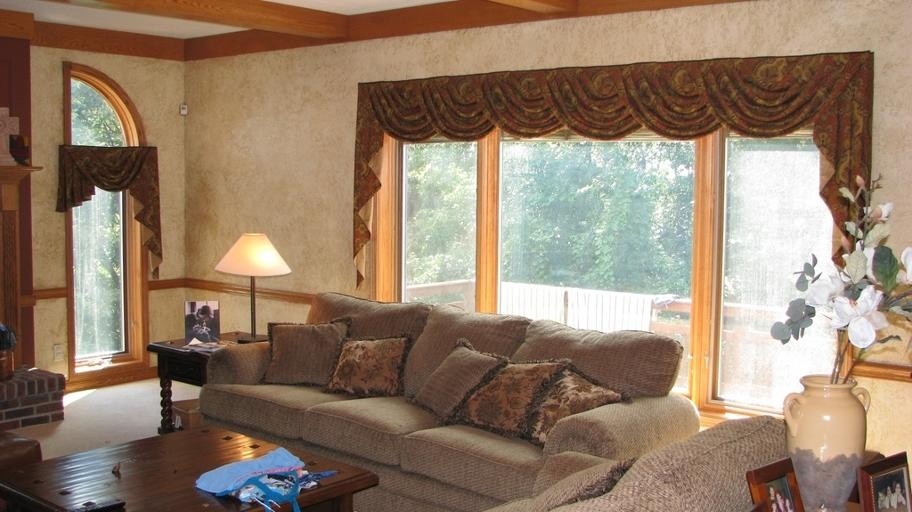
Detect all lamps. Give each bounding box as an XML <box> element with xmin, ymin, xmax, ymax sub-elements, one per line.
<box><xmin>214</xmin><ymin>232</ymin><xmax>293</xmax><ymax>344</ymax></box>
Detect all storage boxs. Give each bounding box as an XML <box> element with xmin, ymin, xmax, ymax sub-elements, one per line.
<box><xmin>169</xmin><ymin>398</ymin><xmax>202</xmax><ymax>430</ymax></box>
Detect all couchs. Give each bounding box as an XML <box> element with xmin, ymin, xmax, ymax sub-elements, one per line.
<box><xmin>197</xmin><ymin>292</ymin><xmax>700</xmax><ymax>511</ymax></box>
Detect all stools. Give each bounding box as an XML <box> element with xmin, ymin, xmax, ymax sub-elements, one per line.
<box><xmin>1</xmin><ymin>429</ymin><xmax>44</xmax><ymax>475</ymax></box>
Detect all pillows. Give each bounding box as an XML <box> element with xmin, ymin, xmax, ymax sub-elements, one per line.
<box><xmin>447</xmin><ymin>353</ymin><xmax>573</xmax><ymax>439</ymax></box>
<box><xmin>408</xmin><ymin>335</ymin><xmax>511</xmax><ymax>422</ymax></box>
<box><xmin>320</xmin><ymin>333</ymin><xmax>413</xmax><ymax>398</ymax></box>
<box><xmin>519</xmin><ymin>451</ymin><xmax>637</xmax><ymax>512</ymax></box>
<box><xmin>522</xmin><ymin>360</ymin><xmax>629</xmax><ymax>449</ymax></box>
<box><xmin>261</xmin><ymin>314</ymin><xmax>352</xmax><ymax>386</ymax></box>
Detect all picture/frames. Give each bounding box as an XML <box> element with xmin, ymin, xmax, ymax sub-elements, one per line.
<box><xmin>746</xmin><ymin>458</ymin><xmax>803</xmax><ymax>512</ymax></box>
<box><xmin>856</xmin><ymin>451</ymin><xmax>912</xmax><ymax>512</ymax></box>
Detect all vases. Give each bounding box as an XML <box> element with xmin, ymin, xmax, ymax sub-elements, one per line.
<box><xmin>783</xmin><ymin>373</ymin><xmax>871</xmax><ymax>512</ymax></box>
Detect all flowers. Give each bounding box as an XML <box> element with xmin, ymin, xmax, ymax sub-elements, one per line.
<box><xmin>772</xmin><ymin>175</ymin><xmax>912</xmax><ymax>384</ymax></box>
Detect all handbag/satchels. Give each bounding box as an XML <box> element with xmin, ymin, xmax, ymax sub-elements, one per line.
<box><xmin>194</xmin><ymin>446</ymin><xmax>305</xmax><ymax>512</ymax></box>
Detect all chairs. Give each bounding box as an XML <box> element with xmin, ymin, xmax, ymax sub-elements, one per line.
<box><xmin>490</xmin><ymin>416</ymin><xmax>787</xmax><ymax>512</ymax></box>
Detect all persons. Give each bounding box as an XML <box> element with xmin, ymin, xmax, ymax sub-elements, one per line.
<box><xmin>875</xmin><ymin>482</ymin><xmax>906</xmax><ymax>511</ymax></box>
<box><xmin>767</xmin><ymin>484</ymin><xmax>792</xmax><ymax>511</ymax></box>
<box><xmin>186</xmin><ymin>305</ymin><xmax>218</xmax><ymax>339</ymax></box>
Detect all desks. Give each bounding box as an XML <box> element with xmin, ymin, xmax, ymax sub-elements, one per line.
<box><xmin>145</xmin><ymin>329</ymin><xmax>272</xmax><ymax>432</ymax></box>
